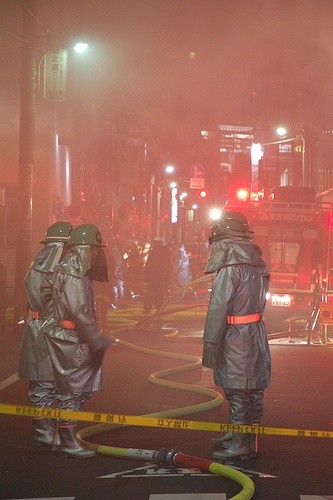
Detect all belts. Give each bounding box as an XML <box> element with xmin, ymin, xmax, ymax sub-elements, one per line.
<box><xmin>55</xmin><ymin>319</ymin><xmax>74</xmax><ymax>329</ymax></box>
<box><xmin>26</xmin><ymin>308</ymin><xmax>39</xmax><ymax>320</ymax></box>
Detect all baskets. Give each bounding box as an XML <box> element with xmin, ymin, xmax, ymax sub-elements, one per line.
<box><xmin>225</xmin><ymin>313</ymin><xmax>260</xmax><ymax>324</ymax></box>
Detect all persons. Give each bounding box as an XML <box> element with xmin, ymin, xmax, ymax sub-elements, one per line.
<box><xmin>42</xmin><ymin>223</ymin><xmax>116</xmax><ymax>459</ymax></box>
<box><xmin>91</xmin><ymin>228</ymin><xmax>211</xmax><ymax>314</ymax></box>
<box><xmin>17</xmin><ymin>222</ymin><xmax>74</xmax><ymax>452</ymax></box>
<box><xmin>202</xmin><ymin>216</ymin><xmax>272</xmax><ymax>464</ymax></box>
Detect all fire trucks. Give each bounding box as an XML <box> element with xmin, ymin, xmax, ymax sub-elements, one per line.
<box><xmin>224</xmin><ymin>185</ymin><xmax>333</xmax><ymax>345</ymax></box>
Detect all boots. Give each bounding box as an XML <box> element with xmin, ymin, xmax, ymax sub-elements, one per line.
<box><xmin>50</xmin><ymin>423</ymin><xmax>97</xmax><ymax>459</ymax></box>
<box><xmin>210</xmin><ymin>421</ymin><xmax>261</xmax><ymax>459</ymax></box>
<box><xmin>31</xmin><ymin>418</ymin><xmax>55</xmax><ymax>444</ymax></box>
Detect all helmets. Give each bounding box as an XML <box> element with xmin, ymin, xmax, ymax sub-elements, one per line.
<box><xmin>68</xmin><ymin>223</ymin><xmax>106</xmax><ymax>247</ymax></box>
<box><xmin>40</xmin><ymin>222</ymin><xmax>74</xmax><ymax>243</ymax></box>
<box><xmin>207</xmin><ymin>211</ymin><xmax>254</xmax><ymax>242</ymax></box>
